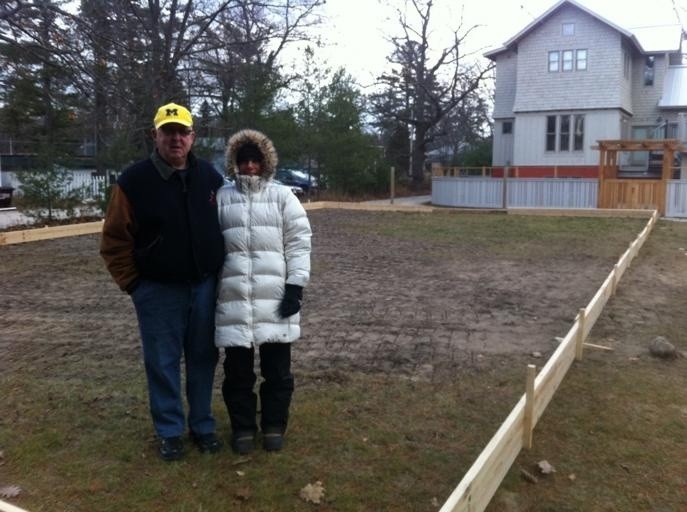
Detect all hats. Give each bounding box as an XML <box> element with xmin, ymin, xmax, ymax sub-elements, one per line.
<box><xmin>153</xmin><ymin>102</ymin><xmax>193</xmax><ymax>131</ymax></box>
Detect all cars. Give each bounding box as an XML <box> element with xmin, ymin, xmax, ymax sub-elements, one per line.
<box><xmin>273</xmin><ymin>168</ymin><xmax>318</xmax><ymax>194</ymax></box>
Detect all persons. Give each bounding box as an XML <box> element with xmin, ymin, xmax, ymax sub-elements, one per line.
<box><xmin>100</xmin><ymin>103</ymin><xmax>224</xmax><ymax>459</ymax></box>
<box><xmin>214</xmin><ymin>129</ymin><xmax>312</xmax><ymax>453</ymax></box>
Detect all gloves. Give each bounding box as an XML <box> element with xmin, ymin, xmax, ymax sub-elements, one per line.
<box><xmin>278</xmin><ymin>282</ymin><xmax>304</xmax><ymax>319</ymax></box>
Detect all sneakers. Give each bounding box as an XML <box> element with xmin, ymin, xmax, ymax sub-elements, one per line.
<box><xmin>232</xmin><ymin>426</ymin><xmax>284</xmax><ymax>455</ymax></box>
<box><xmin>190</xmin><ymin>432</ymin><xmax>225</xmax><ymax>453</ymax></box>
<box><xmin>157</xmin><ymin>437</ymin><xmax>180</xmax><ymax>462</ymax></box>
